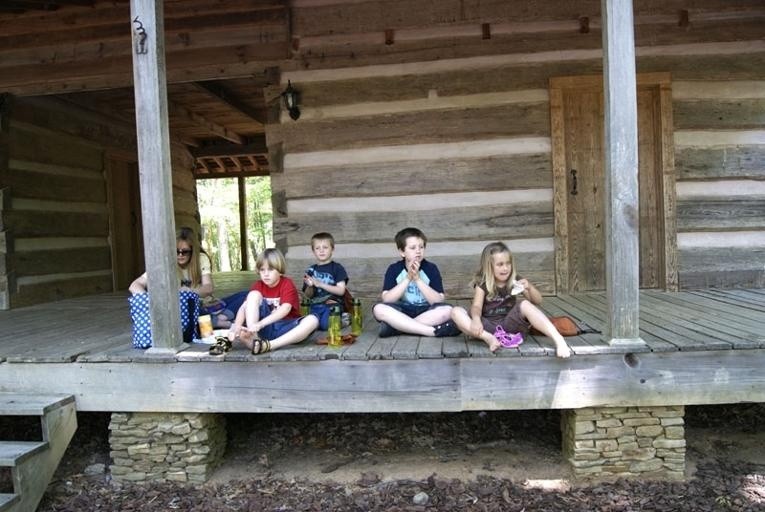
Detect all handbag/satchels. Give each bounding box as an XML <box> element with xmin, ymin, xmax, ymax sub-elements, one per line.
<box><xmin>529</xmin><ymin>316</ymin><xmax>578</xmax><ymax>336</ymax></box>
<box><xmin>127</xmin><ymin>291</ymin><xmax>200</xmax><ymax>348</ymax></box>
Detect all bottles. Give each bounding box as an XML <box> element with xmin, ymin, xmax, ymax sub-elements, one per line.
<box><xmin>326</xmin><ymin>306</ymin><xmax>342</xmax><ymax>346</ymax></box>
<box><xmin>299</xmin><ymin>299</ymin><xmax>307</xmax><ymax>319</ymax></box>
<box><xmin>349</xmin><ymin>297</ymin><xmax>362</xmax><ymax>337</ymax></box>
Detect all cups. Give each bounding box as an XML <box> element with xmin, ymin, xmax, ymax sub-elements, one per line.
<box><xmin>197</xmin><ymin>313</ymin><xmax>215</xmax><ymax>342</ymax></box>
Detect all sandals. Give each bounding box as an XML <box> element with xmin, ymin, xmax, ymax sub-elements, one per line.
<box><xmin>252</xmin><ymin>338</ymin><xmax>270</xmax><ymax>354</ymax></box>
<box><xmin>209</xmin><ymin>337</ymin><xmax>232</xmax><ymax>354</ymax></box>
<box><xmin>493</xmin><ymin>325</ymin><xmax>523</xmax><ymax>348</ymax></box>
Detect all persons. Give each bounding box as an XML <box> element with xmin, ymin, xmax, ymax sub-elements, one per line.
<box><xmin>126</xmin><ymin>227</ymin><xmax>249</xmax><ymax>332</ymax></box>
<box><xmin>451</xmin><ymin>241</ymin><xmax>571</xmax><ymax>358</ymax></box>
<box><xmin>373</xmin><ymin>229</ymin><xmax>463</xmax><ymax>338</ymax></box>
<box><xmin>228</xmin><ymin>247</ymin><xmax>320</xmax><ymax>354</ymax></box>
<box><xmin>302</xmin><ymin>230</ymin><xmax>354</xmax><ymax>330</ymax></box>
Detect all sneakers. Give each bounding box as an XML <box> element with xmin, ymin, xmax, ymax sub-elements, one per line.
<box><xmin>378</xmin><ymin>321</ymin><xmax>398</xmax><ymax>337</ymax></box>
<box><xmin>433</xmin><ymin>321</ymin><xmax>461</xmax><ymax>337</ymax></box>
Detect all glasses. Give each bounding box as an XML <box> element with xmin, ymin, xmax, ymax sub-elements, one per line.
<box><xmin>177</xmin><ymin>249</ymin><xmax>191</xmax><ymax>257</ymax></box>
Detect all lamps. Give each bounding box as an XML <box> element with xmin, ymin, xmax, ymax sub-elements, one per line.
<box><xmin>282</xmin><ymin>79</ymin><xmax>300</xmax><ymax>121</ymax></box>
